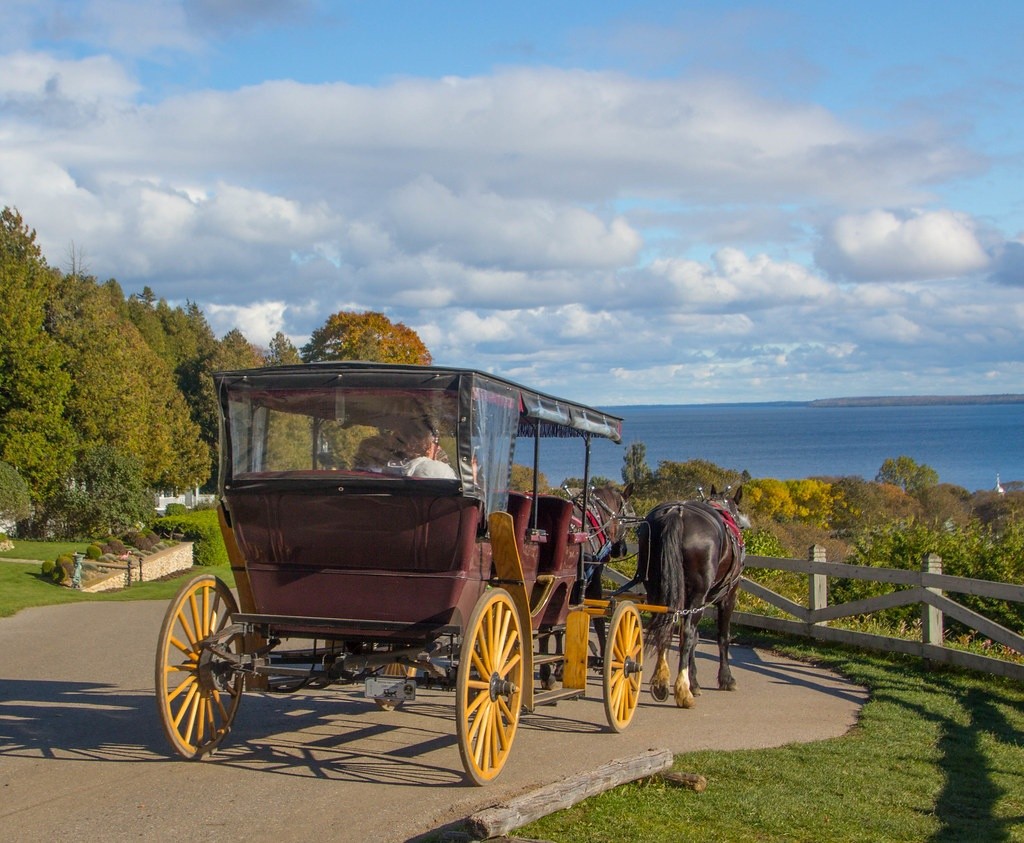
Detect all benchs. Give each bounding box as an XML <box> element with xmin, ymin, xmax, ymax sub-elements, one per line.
<box><xmin>227</xmin><ymin>471</ymin><xmax>573</xmax><ymax>626</ymax></box>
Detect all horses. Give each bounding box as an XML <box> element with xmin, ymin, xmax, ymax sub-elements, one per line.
<box><xmin>537</xmin><ymin>481</ymin><xmax>744</xmax><ymax>708</ymax></box>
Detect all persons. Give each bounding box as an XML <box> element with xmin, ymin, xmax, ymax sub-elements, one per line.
<box><xmin>386</xmin><ymin>419</ymin><xmax>456</xmax><ymax>479</ymax></box>
<box><xmin>351</xmin><ymin>436</ymin><xmax>389</xmax><ymax>472</ymax></box>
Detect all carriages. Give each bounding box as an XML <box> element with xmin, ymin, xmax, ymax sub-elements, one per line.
<box><xmin>152</xmin><ymin>363</ymin><xmax>752</xmax><ymax>787</ymax></box>
